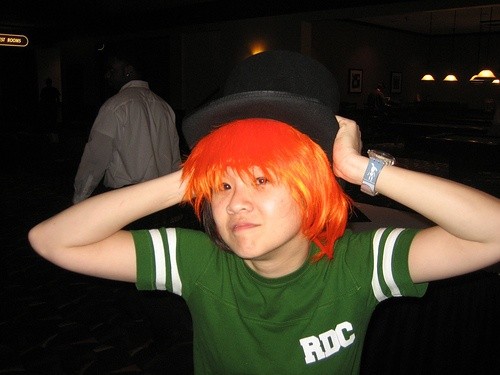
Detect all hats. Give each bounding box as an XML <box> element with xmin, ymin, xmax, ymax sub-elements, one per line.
<box><xmin>181</xmin><ymin>49</ymin><xmax>343</xmax><ymax>168</ymax></box>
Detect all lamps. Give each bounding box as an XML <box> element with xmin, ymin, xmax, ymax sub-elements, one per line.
<box><xmin>420</xmin><ymin>8</ymin><xmax>500</xmax><ymax>85</ymax></box>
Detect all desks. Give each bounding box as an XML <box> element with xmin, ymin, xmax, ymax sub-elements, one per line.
<box><xmin>388</xmin><ymin>120</ymin><xmax>491</xmax><ymax>151</ymax></box>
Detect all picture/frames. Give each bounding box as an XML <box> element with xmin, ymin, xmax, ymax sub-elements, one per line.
<box><xmin>390</xmin><ymin>71</ymin><xmax>403</xmax><ymax>93</ymax></box>
<box><xmin>348</xmin><ymin>68</ymin><xmax>363</xmax><ymax>92</ymax></box>
